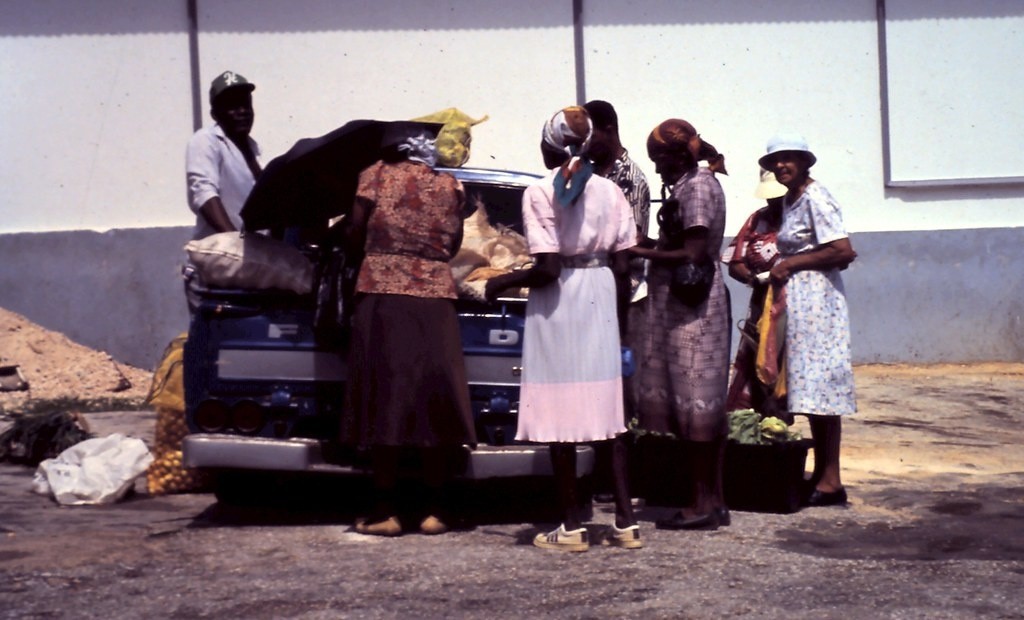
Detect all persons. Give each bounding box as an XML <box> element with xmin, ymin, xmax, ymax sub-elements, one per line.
<box><xmin>621</xmin><ymin>118</ymin><xmax>731</xmax><ymax>533</ymax></box>
<box><xmin>481</xmin><ymin>106</ymin><xmax>642</xmax><ymax>550</ymax></box>
<box><xmin>185</xmin><ymin>70</ymin><xmax>265</xmax><ymax>243</ymax></box>
<box><xmin>757</xmin><ymin>135</ymin><xmax>857</xmax><ymax>504</ymax></box>
<box><xmin>726</xmin><ymin>166</ymin><xmax>795</xmax><ymax>425</ymax></box>
<box><xmin>339</xmin><ymin>134</ymin><xmax>466</xmax><ymax>532</ymax></box>
<box><xmin>573</xmin><ymin>100</ymin><xmax>651</xmax><ymax>298</ymax></box>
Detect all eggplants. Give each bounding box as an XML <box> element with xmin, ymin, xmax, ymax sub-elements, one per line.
<box><xmin>437</xmin><ymin>121</ymin><xmax>471</xmax><ymax>167</ymax></box>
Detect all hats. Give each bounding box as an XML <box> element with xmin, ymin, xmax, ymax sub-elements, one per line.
<box><xmin>210</xmin><ymin>71</ymin><xmax>254</xmax><ymax>103</ymax></box>
<box><xmin>758</xmin><ymin>139</ymin><xmax>816</xmax><ymax>167</ymax></box>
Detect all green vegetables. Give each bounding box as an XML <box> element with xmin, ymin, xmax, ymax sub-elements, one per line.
<box><xmin>726</xmin><ymin>409</ymin><xmax>801</xmax><ymax>445</ymax></box>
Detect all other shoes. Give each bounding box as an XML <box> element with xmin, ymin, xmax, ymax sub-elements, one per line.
<box><xmin>654</xmin><ymin>505</ymin><xmax>730</xmax><ymax>532</ymax></box>
<box><xmin>805</xmin><ymin>479</ymin><xmax>847</xmax><ymax>507</ymax></box>
<box><xmin>357</xmin><ymin>516</ymin><xmax>400</xmax><ymax>534</ymax></box>
<box><xmin>420</xmin><ymin>515</ymin><xmax>447</xmax><ymax>532</ymax></box>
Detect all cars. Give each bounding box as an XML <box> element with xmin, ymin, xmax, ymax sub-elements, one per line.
<box><xmin>183</xmin><ymin>168</ymin><xmax>598</xmax><ymax>513</ymax></box>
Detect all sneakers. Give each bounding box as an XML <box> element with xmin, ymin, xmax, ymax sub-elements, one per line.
<box><xmin>595</xmin><ymin>521</ymin><xmax>641</xmax><ymax>549</ymax></box>
<box><xmin>533</xmin><ymin>524</ymin><xmax>589</xmax><ymax>552</ymax></box>
